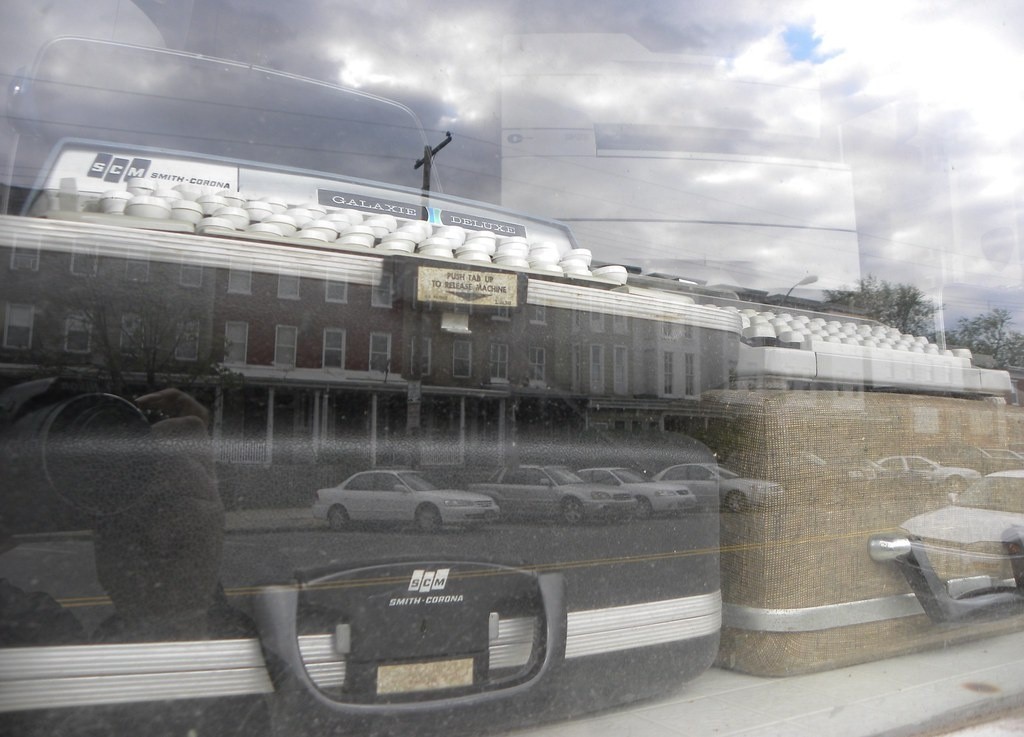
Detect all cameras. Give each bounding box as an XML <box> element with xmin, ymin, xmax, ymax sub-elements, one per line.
<box><xmin>0</xmin><ymin>376</ymin><xmax>178</xmax><ymax>533</ymax></box>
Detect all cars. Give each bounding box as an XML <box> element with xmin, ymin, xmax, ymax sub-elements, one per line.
<box><xmin>467</xmin><ymin>464</ymin><xmax>639</xmax><ymax>527</ymax></box>
<box><xmin>786</xmin><ymin>441</ymin><xmax>1023</xmax><ymax>503</ymax></box>
<box><xmin>310</xmin><ymin>468</ymin><xmax>500</xmax><ymax>535</ymax></box>
<box><xmin>575</xmin><ymin>465</ymin><xmax>696</xmax><ymax>520</ymax></box>
<box><xmin>652</xmin><ymin>462</ymin><xmax>785</xmax><ymax>514</ymax></box>
<box><xmin>890</xmin><ymin>469</ymin><xmax>1023</xmax><ymax>580</ymax></box>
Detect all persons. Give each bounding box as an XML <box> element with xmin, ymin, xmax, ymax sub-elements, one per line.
<box><xmin>0</xmin><ymin>388</ymin><xmax>260</xmax><ymax>737</ymax></box>
